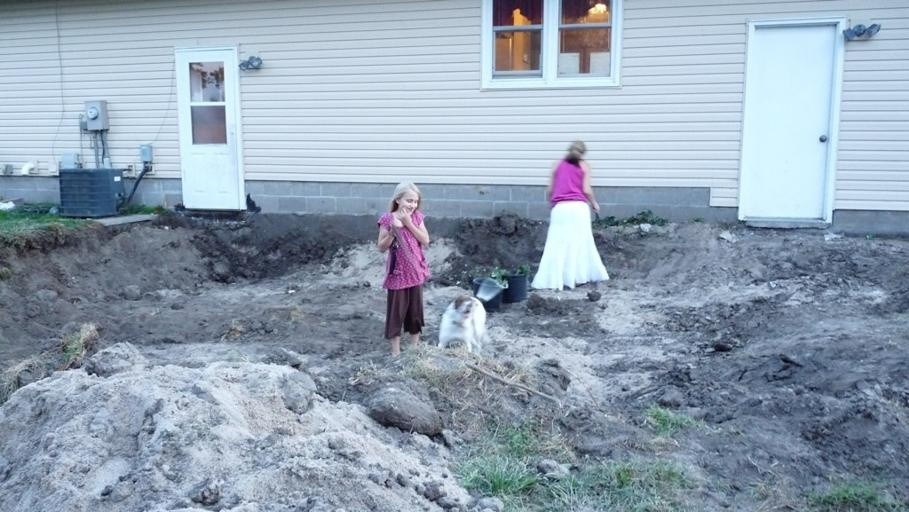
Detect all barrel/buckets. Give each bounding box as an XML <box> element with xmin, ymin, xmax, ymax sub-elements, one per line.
<box><xmin>473</xmin><ymin>277</ymin><xmax>504</xmax><ymax>312</ymax></box>
<box><xmin>502</xmin><ymin>274</ymin><xmax>526</xmax><ymax>302</ymax></box>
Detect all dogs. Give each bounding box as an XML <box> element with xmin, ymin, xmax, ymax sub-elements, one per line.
<box><xmin>436</xmin><ymin>294</ymin><xmax>487</xmax><ymax>354</ymax></box>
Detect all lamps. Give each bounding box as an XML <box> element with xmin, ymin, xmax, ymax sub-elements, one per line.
<box><xmin>239</xmin><ymin>56</ymin><xmax>262</xmax><ymax>70</ymax></box>
<box><xmin>843</xmin><ymin>23</ymin><xmax>881</xmax><ymax>41</ymax></box>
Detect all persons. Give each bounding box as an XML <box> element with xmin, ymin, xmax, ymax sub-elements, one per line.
<box><xmin>530</xmin><ymin>139</ymin><xmax>610</xmax><ymax>292</ymax></box>
<box><xmin>376</xmin><ymin>177</ymin><xmax>434</xmax><ymax>358</ymax></box>
